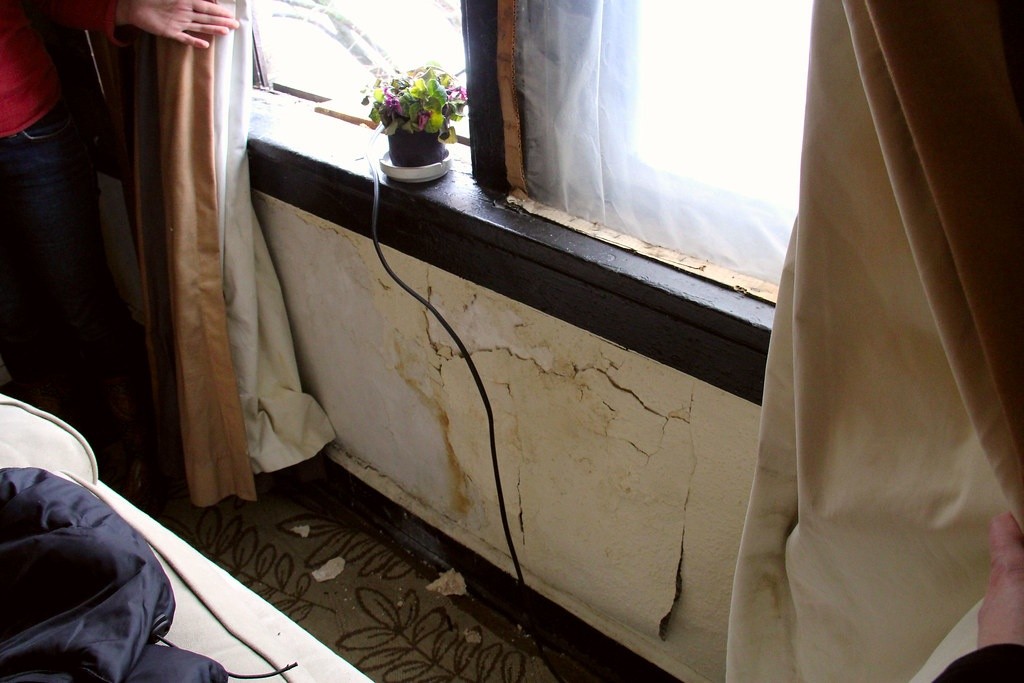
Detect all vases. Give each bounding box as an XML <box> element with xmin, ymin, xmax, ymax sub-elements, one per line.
<box><xmin>379</xmin><ymin>115</ymin><xmax>448</xmax><ymax>168</ymax></box>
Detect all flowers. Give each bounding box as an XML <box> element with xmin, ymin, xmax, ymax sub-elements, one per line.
<box><xmin>364</xmin><ymin>62</ymin><xmax>468</xmax><ymax>144</ymax></box>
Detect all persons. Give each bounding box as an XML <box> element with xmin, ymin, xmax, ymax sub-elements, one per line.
<box><xmin>0</xmin><ymin>0</ymin><xmax>239</xmax><ymax>422</ymax></box>
